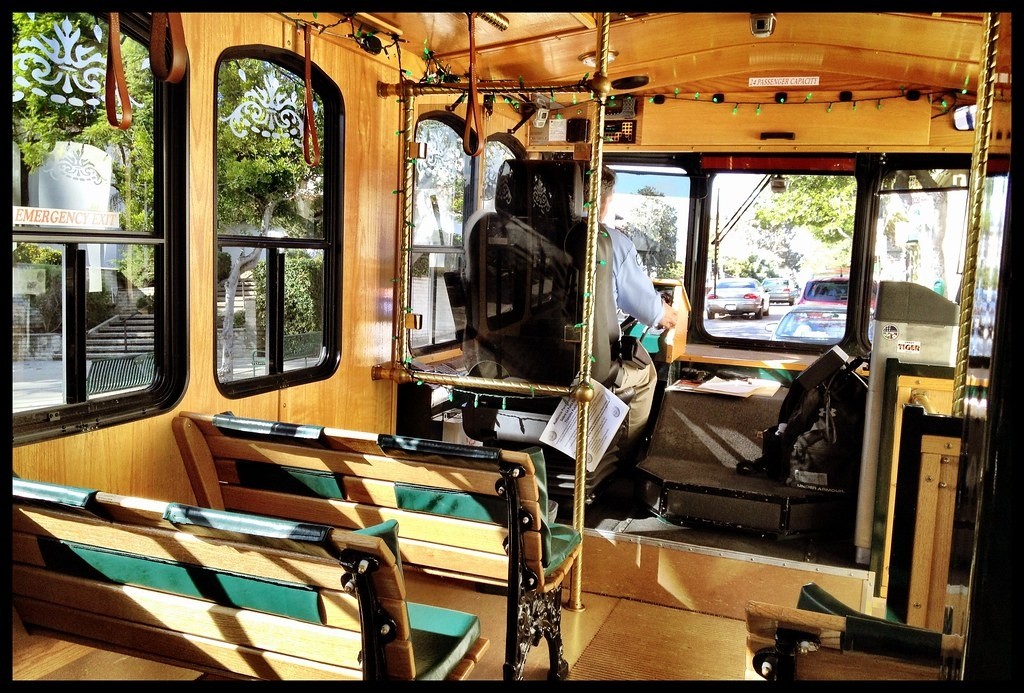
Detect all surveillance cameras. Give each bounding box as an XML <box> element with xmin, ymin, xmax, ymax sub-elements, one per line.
<box><xmin>749</xmin><ymin>12</ymin><xmax>777</xmax><ymax>38</ymax></box>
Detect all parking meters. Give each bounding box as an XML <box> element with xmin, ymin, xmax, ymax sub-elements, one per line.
<box><xmin>934</xmin><ymin>280</ymin><xmax>945</xmax><ymax>297</ymax></box>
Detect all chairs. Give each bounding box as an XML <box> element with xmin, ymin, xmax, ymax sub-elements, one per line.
<box><xmin>744</xmin><ymin>583</ymin><xmax>965</xmax><ymax>681</ymax></box>
<box><xmin>459</xmin><ymin>157</ymin><xmax>634</xmax><ymax>521</ymax></box>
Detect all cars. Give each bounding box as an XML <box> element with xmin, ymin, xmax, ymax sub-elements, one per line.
<box><xmin>705</xmin><ymin>277</ymin><xmax>770</xmax><ymax>320</ymax></box>
<box><xmin>761</xmin><ymin>278</ymin><xmax>797</xmax><ymax>306</ymax></box>
<box><xmin>766</xmin><ymin>278</ymin><xmax>878</xmax><ymax>348</ymax></box>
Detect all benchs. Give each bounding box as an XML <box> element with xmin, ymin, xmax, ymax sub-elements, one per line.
<box><xmin>174</xmin><ymin>408</ymin><xmax>584</xmax><ymax>681</ymax></box>
<box><xmin>12</xmin><ymin>476</ymin><xmax>490</xmax><ymax>680</ymax></box>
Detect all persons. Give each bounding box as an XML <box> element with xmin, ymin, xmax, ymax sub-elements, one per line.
<box><xmin>582</xmin><ymin>161</ymin><xmax>679</xmax><ymax>444</ymax></box>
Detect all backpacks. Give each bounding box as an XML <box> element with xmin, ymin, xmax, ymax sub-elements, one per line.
<box><xmin>736</xmin><ymin>344</ymin><xmax>868</xmax><ymax>488</ymax></box>
